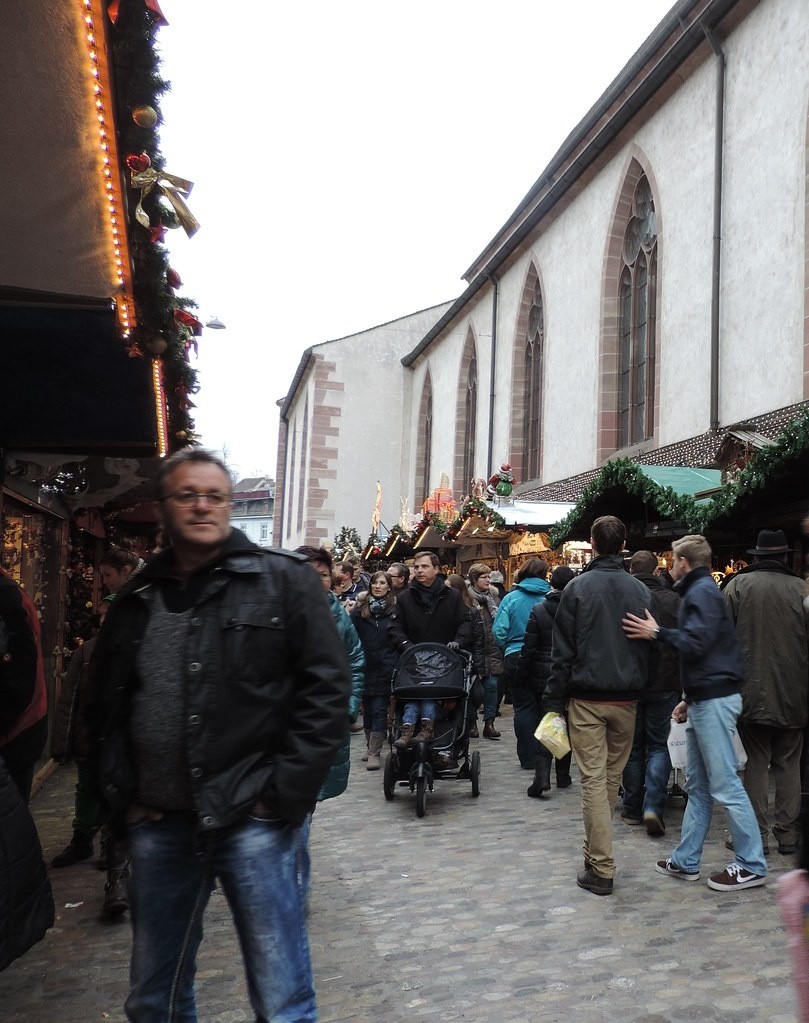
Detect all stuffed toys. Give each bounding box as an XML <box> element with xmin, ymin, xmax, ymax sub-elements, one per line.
<box><xmin>83</xmin><ymin>563</ymin><xmax>93</xmax><ymax>581</ymax></box>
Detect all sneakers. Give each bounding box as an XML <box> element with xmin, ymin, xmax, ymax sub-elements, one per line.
<box><xmin>707</xmin><ymin>863</ymin><xmax>765</xmax><ymax>891</ymax></box>
<box><xmin>656</xmin><ymin>858</ymin><xmax>700</xmax><ymax>881</ymax></box>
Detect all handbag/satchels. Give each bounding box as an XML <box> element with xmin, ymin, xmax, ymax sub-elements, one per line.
<box><xmin>534</xmin><ymin>711</ymin><xmax>571</xmax><ymax>760</ymax></box>
<box><xmin>733</xmin><ymin>729</ymin><xmax>748</xmax><ymax>771</ymax></box>
<box><xmin>668</xmin><ymin>716</ymin><xmax>688</xmax><ymax>767</ymax></box>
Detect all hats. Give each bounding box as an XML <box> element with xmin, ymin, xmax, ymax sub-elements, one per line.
<box><xmin>489</xmin><ymin>571</ymin><xmax>504</xmax><ymax>583</ymax></box>
<box><xmin>746</xmin><ymin>530</ymin><xmax>797</xmax><ymax>555</ymax></box>
<box><xmin>550</xmin><ymin>566</ymin><xmax>575</xmax><ymax>589</ymax></box>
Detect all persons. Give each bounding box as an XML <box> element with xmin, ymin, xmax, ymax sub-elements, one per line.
<box><xmin>0</xmin><ymin>569</ymin><xmax>48</xmax><ymax>802</ymax></box>
<box><xmin>331</xmin><ymin>551</ymin><xmax>573</xmax><ymax>797</ymax></box>
<box><xmin>51</xmin><ymin>544</ymin><xmax>147</xmax><ymax>915</ymax></box>
<box><xmin>542</xmin><ymin>516</ymin><xmax>662</xmax><ymax>897</ymax></box>
<box><xmin>486</xmin><ymin>463</ymin><xmax>516</xmax><ymax>501</ymax></box>
<box><xmin>93</xmin><ymin>446</ymin><xmax>351</xmax><ymax>1023</ymax></box>
<box><xmin>629</xmin><ymin>528</ymin><xmax>809</xmax><ymax>856</ymax></box>
<box><xmin>290</xmin><ymin>545</ymin><xmax>365</xmax><ymax>912</ymax></box>
<box><xmin>621</xmin><ymin>534</ymin><xmax>767</xmax><ymax>892</ymax></box>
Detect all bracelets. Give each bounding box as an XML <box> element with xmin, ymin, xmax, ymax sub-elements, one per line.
<box><xmin>652</xmin><ymin>628</ymin><xmax>659</xmax><ymax>639</ymax></box>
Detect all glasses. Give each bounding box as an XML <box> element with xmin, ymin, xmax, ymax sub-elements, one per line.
<box><xmin>389</xmin><ymin>573</ymin><xmax>401</xmax><ymax>577</ymax></box>
<box><xmin>163</xmin><ymin>492</ymin><xmax>233</xmax><ymax>509</ymax></box>
<box><xmin>479</xmin><ymin>575</ymin><xmax>490</xmax><ymax>580</ymax></box>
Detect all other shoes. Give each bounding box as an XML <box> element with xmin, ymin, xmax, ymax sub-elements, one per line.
<box><xmin>351</xmin><ymin>722</ymin><xmax>362</xmax><ymax>732</ymax></box>
<box><xmin>584</xmin><ymin>860</ymin><xmax>592</xmax><ymax>870</ymax></box>
<box><xmin>621</xmin><ymin>809</ymin><xmax>666</xmax><ymax>836</ymax></box>
<box><xmin>725</xmin><ymin>833</ymin><xmax>796</xmax><ymax>854</ymax></box>
<box><xmin>575</xmin><ymin>868</ymin><xmax>613</xmax><ymax>894</ymax></box>
<box><xmin>98</xmin><ymin>850</ymin><xmax>108</xmax><ymax>868</ymax></box>
<box><xmin>53</xmin><ymin>840</ymin><xmax>94</xmax><ymax>866</ymax></box>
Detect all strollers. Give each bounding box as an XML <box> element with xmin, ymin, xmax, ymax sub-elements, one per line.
<box><xmin>383</xmin><ymin>642</ymin><xmax>482</xmax><ymax>819</ymax></box>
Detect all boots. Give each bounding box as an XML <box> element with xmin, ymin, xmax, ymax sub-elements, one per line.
<box><xmin>410</xmin><ymin>720</ymin><xmax>434</xmax><ymax>745</ymax></box>
<box><xmin>367</xmin><ymin>732</ymin><xmax>385</xmax><ymax>770</ymax></box>
<box><xmin>363</xmin><ymin>728</ymin><xmax>372</xmax><ymax>761</ymax></box>
<box><xmin>556</xmin><ymin>751</ymin><xmax>573</xmax><ymax>788</ymax></box>
<box><xmin>528</xmin><ymin>755</ymin><xmax>552</xmax><ymax>796</ymax></box>
<box><xmin>484</xmin><ymin>719</ymin><xmax>501</xmax><ymax>737</ymax></box>
<box><xmin>103</xmin><ymin>872</ymin><xmax>129</xmax><ymax>916</ymax></box>
<box><xmin>393</xmin><ymin>723</ymin><xmax>415</xmax><ymax>749</ymax></box>
<box><xmin>468</xmin><ymin>720</ymin><xmax>480</xmax><ymax>737</ymax></box>
